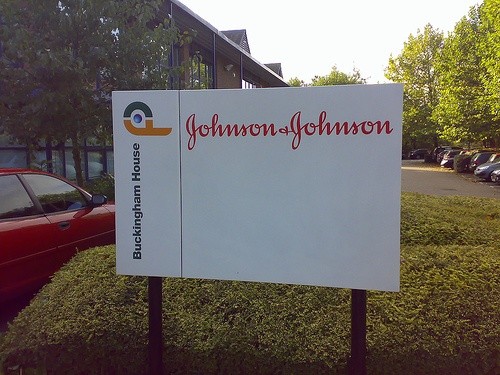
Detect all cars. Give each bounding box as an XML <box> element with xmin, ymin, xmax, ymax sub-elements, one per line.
<box><xmin>408</xmin><ymin>146</ymin><xmax>500</xmax><ymax>182</ymax></box>
<box><xmin>0</xmin><ymin>167</ymin><xmax>115</xmax><ymax>338</ymax></box>
